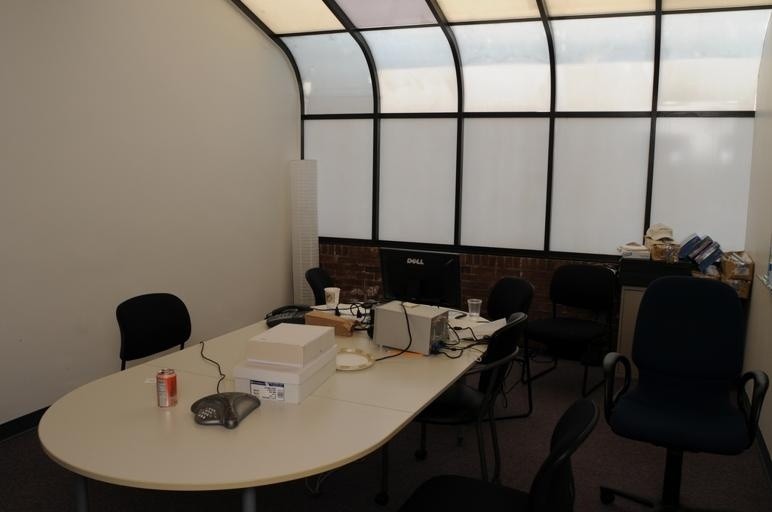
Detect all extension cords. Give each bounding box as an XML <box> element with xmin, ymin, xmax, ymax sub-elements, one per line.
<box><xmin>336</xmin><ymin>313</ymin><xmax>367</xmax><ymax>328</ymax></box>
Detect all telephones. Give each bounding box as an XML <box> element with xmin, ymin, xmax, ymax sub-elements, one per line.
<box><xmin>191</xmin><ymin>392</ymin><xmax>260</xmax><ymax>429</ymax></box>
<box><xmin>264</xmin><ymin>304</ymin><xmax>313</xmax><ymax>328</ymax></box>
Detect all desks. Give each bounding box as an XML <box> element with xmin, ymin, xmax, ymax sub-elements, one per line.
<box><xmin>37</xmin><ymin>304</ymin><xmax>503</xmax><ymax>512</ymax></box>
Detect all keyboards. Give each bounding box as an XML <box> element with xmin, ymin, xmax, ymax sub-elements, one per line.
<box><xmin>403</xmin><ymin>302</ymin><xmax>467</xmax><ymax>320</ymax></box>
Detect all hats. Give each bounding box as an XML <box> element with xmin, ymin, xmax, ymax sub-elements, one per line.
<box><xmin>647</xmin><ymin>223</ymin><xmax>675</xmax><ymax>242</ymax></box>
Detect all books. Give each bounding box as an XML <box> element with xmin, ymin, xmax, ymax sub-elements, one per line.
<box><xmin>616</xmin><ymin>231</ymin><xmax>726</xmax><ymax>274</ymax></box>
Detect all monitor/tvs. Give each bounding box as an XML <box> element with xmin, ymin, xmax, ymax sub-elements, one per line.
<box><xmin>379</xmin><ymin>247</ymin><xmax>462</xmax><ymax>311</ymax></box>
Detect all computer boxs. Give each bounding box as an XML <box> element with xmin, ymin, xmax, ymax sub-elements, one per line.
<box><xmin>375</xmin><ymin>298</ymin><xmax>449</xmax><ymax>356</ymax></box>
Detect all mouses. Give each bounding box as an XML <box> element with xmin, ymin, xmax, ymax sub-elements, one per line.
<box><xmin>361</xmin><ymin>300</ymin><xmax>377</xmax><ymax>308</ymax></box>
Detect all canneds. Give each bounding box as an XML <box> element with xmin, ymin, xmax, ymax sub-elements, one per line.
<box><xmin>157</xmin><ymin>368</ymin><xmax>178</xmax><ymax>408</ymax></box>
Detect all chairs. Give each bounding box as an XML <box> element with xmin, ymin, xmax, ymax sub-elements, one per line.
<box><xmin>395</xmin><ymin>397</ymin><xmax>600</xmax><ymax>512</ymax></box>
<box><xmin>375</xmin><ymin>313</ymin><xmax>529</xmax><ymax>506</ymax></box>
<box><xmin>305</xmin><ymin>267</ymin><xmax>333</xmax><ymax>305</ymax></box>
<box><xmin>481</xmin><ymin>277</ymin><xmax>535</xmax><ymax>422</ymax></box>
<box><xmin>522</xmin><ymin>264</ymin><xmax>617</xmax><ymax>398</ymax></box>
<box><xmin>599</xmin><ymin>275</ymin><xmax>769</xmax><ymax>512</ymax></box>
<box><xmin>116</xmin><ymin>293</ymin><xmax>192</xmax><ymax>371</ymax></box>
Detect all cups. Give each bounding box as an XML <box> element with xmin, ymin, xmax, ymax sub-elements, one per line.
<box><xmin>468</xmin><ymin>298</ymin><xmax>483</xmax><ymax>318</ymax></box>
<box><xmin>324</xmin><ymin>287</ymin><xmax>340</xmax><ymax>306</ymax></box>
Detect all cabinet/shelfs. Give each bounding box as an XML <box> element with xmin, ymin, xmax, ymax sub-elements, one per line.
<box><xmin>616</xmin><ymin>285</ymin><xmax>646</xmax><ymax>380</ymax></box>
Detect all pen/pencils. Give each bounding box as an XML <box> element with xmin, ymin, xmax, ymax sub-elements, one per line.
<box><xmin>455</xmin><ymin>314</ymin><xmax>467</xmax><ymax>319</ymax></box>
